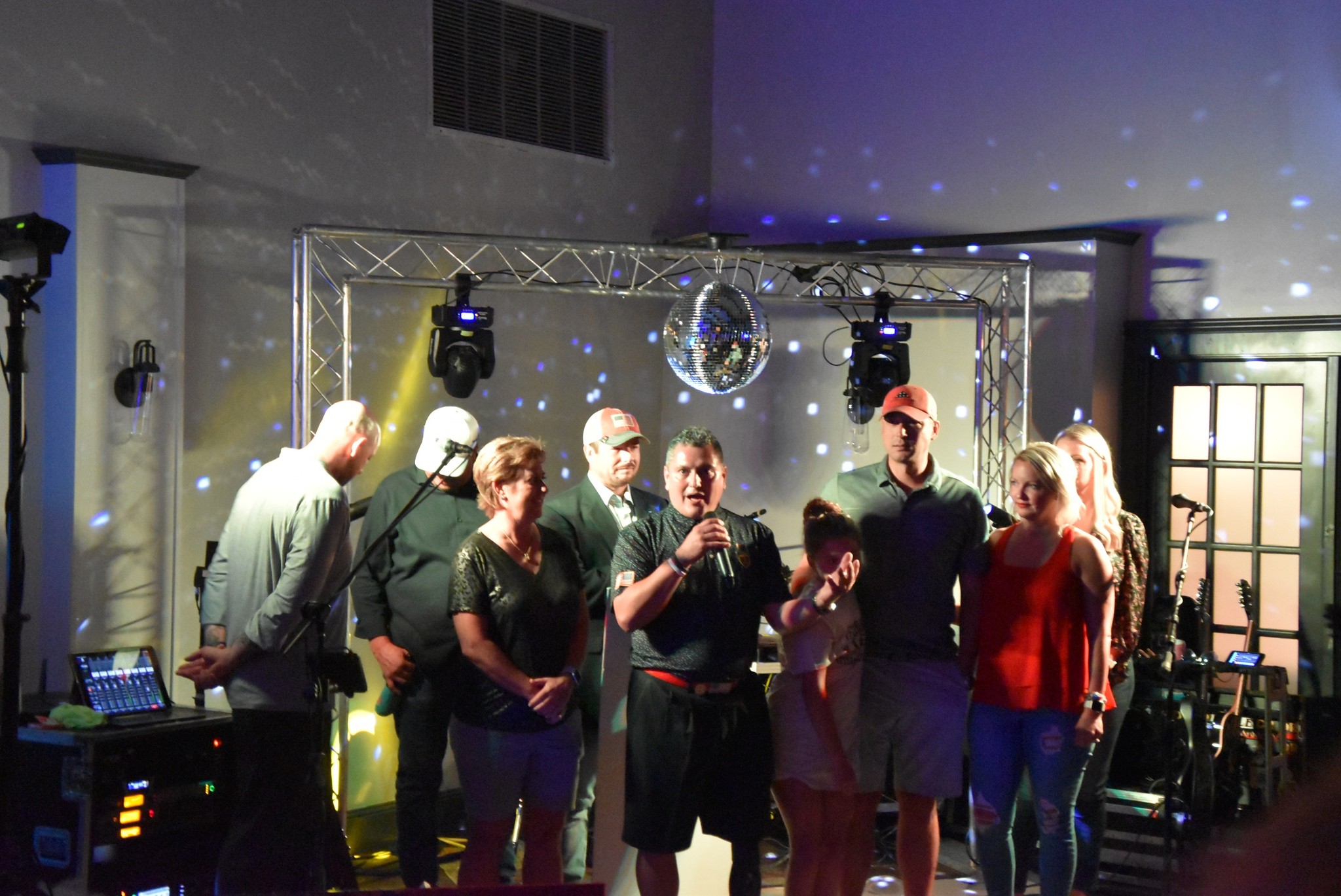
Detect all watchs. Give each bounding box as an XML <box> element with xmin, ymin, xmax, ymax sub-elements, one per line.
<box><xmin>810</xmin><ymin>594</ymin><xmax>837</xmax><ymax>616</ymax></box>
<box><xmin>1084</xmin><ymin>700</ymin><xmax>1106</xmax><ymax>714</ymax></box>
<box><xmin>560</xmin><ymin>666</ymin><xmax>582</xmax><ymax>688</ymax></box>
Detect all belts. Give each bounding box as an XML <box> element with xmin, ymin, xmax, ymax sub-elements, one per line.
<box><xmin>644</xmin><ymin>668</ymin><xmax>743</xmax><ymax>694</ymax></box>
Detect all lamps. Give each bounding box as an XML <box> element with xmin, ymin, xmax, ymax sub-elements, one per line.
<box><xmin>114</xmin><ymin>337</ymin><xmax>161</xmax><ymax>445</ymax></box>
<box><xmin>426</xmin><ymin>271</ymin><xmax>494</xmax><ymax>397</ymax></box>
<box><xmin>843</xmin><ymin>398</ymin><xmax>876</xmax><ymax>451</ymax></box>
<box><xmin>844</xmin><ymin>290</ymin><xmax>913</xmax><ymax>409</ymax></box>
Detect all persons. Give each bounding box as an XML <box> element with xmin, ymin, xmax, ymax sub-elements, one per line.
<box><xmin>609</xmin><ymin>427</ymin><xmax>862</xmax><ymax>896</ymax></box>
<box><xmin>175</xmin><ymin>400</ymin><xmax>385</xmax><ymax>896</ymax></box>
<box><xmin>449</xmin><ymin>434</ymin><xmax>590</xmax><ymax>886</ymax></box>
<box><xmin>350</xmin><ymin>406</ymin><xmax>495</xmax><ymax>889</ymax></box>
<box><xmin>538</xmin><ymin>408</ymin><xmax>669</xmax><ymax>896</ymax></box>
<box><xmin>789</xmin><ymin>387</ymin><xmax>990</xmax><ymax>896</ymax></box>
<box><xmin>952</xmin><ymin>441</ymin><xmax>1116</xmax><ymax>896</ymax></box>
<box><xmin>1013</xmin><ymin>423</ymin><xmax>1149</xmax><ymax>896</ymax></box>
<box><xmin>772</xmin><ymin>497</ymin><xmax>863</xmax><ymax>896</ymax></box>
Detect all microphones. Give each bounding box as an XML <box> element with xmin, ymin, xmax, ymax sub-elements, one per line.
<box><xmin>442</xmin><ymin>440</ymin><xmax>474</xmax><ymax>457</ymax></box>
<box><xmin>1172</xmin><ymin>494</ymin><xmax>1212</xmax><ymax>512</ymax></box>
<box><xmin>703</xmin><ymin>510</ymin><xmax>735</xmax><ymax>589</ymax></box>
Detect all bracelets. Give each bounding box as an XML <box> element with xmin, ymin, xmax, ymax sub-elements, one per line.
<box><xmin>667</xmin><ymin>554</ymin><xmax>689</xmax><ymax>578</ymax></box>
<box><xmin>1084</xmin><ymin>690</ymin><xmax>1108</xmax><ymax>703</ymax></box>
<box><xmin>204</xmin><ymin>642</ymin><xmax>226</xmax><ymax>648</ymax></box>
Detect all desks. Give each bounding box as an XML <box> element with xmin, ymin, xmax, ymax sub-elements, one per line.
<box><xmin>1212</xmin><ymin>664</ymin><xmax>1290</xmax><ymax>813</ymax></box>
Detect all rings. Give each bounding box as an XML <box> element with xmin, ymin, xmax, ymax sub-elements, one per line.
<box><xmin>558</xmin><ymin>714</ymin><xmax>563</xmax><ymax>720</ymax></box>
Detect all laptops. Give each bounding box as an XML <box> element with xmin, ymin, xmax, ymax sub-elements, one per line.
<box><xmin>68</xmin><ymin>645</ymin><xmax>206</xmax><ymax>727</ymax></box>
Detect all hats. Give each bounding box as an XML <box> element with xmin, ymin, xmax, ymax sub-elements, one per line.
<box><xmin>414</xmin><ymin>406</ymin><xmax>480</xmax><ymax>478</ymax></box>
<box><xmin>879</xmin><ymin>384</ymin><xmax>939</xmax><ymax>425</ymax></box>
<box><xmin>583</xmin><ymin>407</ymin><xmax>652</xmax><ymax>448</ymax></box>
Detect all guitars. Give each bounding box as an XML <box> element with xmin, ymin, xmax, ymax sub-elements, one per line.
<box><xmin>1193</xmin><ymin>578</ymin><xmax>1209</xmax><ymax>701</ymax></box>
<box><xmin>1214</xmin><ymin>580</ymin><xmax>1258</xmax><ymax>817</ymax></box>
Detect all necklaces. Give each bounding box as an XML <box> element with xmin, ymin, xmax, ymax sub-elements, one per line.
<box><xmin>497</xmin><ymin>520</ymin><xmax>533</xmax><ymax>563</ymax></box>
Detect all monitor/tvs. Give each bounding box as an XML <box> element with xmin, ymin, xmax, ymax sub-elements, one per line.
<box><xmin>1226</xmin><ymin>650</ymin><xmax>1265</xmax><ymax>667</ymax></box>
